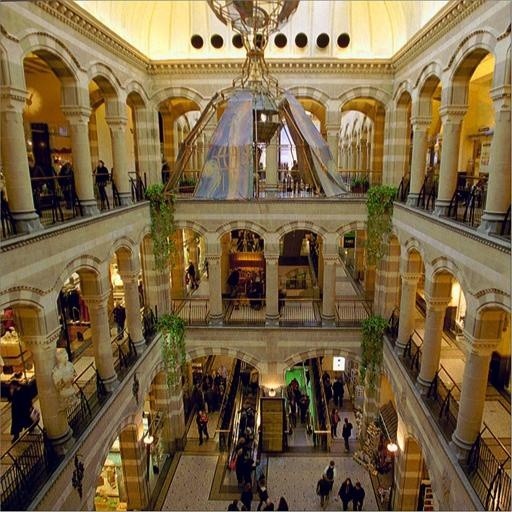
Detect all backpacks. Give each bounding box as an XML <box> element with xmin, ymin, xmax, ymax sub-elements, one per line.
<box><xmin>327</xmin><ymin>466</ymin><xmax>333</xmax><ymax>479</ymax></box>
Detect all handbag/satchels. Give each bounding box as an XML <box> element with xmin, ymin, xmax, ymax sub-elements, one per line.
<box><xmin>29</xmin><ymin>408</ymin><xmax>40</xmax><ymax>425</ymax></box>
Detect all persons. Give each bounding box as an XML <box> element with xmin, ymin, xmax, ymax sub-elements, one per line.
<box><xmin>185</xmin><ymin>361</ymin><xmax>226</xmax><ymax>444</ymax></box>
<box><xmin>228</xmin><ymin>367</ymin><xmax>260</xmax><ymax>485</ymax></box>
<box><xmin>227</xmin><ymin>230</ymin><xmax>266</xmax><ymax>311</ymax></box>
<box><xmin>28</xmin><ymin>152</ymin><xmax>47</xmax><ymax>218</ymax></box>
<box><xmin>228</xmin><ymin>483</ymin><xmax>288</xmax><ymax>512</ymax></box>
<box><xmin>93</xmin><ymin>160</ymin><xmax>111</xmax><ymax>210</ymax></box>
<box><xmin>7</xmin><ymin>379</ymin><xmax>37</xmax><ymax>442</ymax></box>
<box><xmin>57</xmin><ymin>163</ymin><xmax>76</xmax><ymax>210</ymax></box>
<box><xmin>55</xmin><ymin>281</ymin><xmax>148</xmax><ymax>362</ymax></box>
<box><xmin>322</xmin><ymin>370</ymin><xmax>353</xmax><ymax>451</ymax></box>
<box><xmin>291</xmin><ymin>164</ymin><xmax>302</xmax><ymax>194</ymax></box>
<box><xmin>185</xmin><ymin>258</ymin><xmax>209</xmax><ymax>288</ymax></box>
<box><xmin>287</xmin><ymin>377</ymin><xmax>311</xmax><ymax>426</ymax></box>
<box><xmin>317</xmin><ymin>461</ymin><xmax>364</xmax><ymax>511</ymax></box>
<box><xmin>461</xmin><ymin>175</ymin><xmax>488</xmax><ymax>207</ymax></box>
